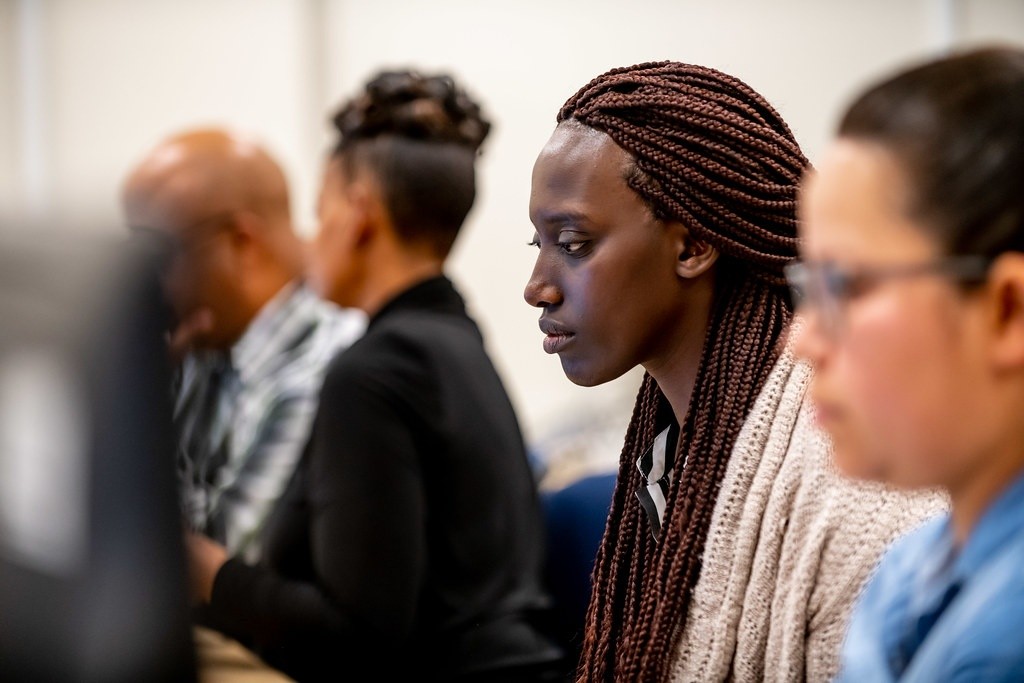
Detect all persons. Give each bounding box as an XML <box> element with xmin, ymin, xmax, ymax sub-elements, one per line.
<box><xmin>781</xmin><ymin>41</ymin><xmax>1024</xmax><ymax>682</ymax></box>
<box><xmin>523</xmin><ymin>61</ymin><xmax>954</xmax><ymax>683</ymax></box>
<box><xmin>122</xmin><ymin>71</ymin><xmax>578</xmax><ymax>683</ymax></box>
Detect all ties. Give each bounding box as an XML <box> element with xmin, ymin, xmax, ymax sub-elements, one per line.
<box><xmin>185</xmin><ymin>349</ymin><xmax>231</xmax><ymax>485</ymax></box>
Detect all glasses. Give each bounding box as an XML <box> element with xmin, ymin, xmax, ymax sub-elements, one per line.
<box><xmin>783</xmin><ymin>254</ymin><xmax>943</xmax><ymax>316</ymax></box>
